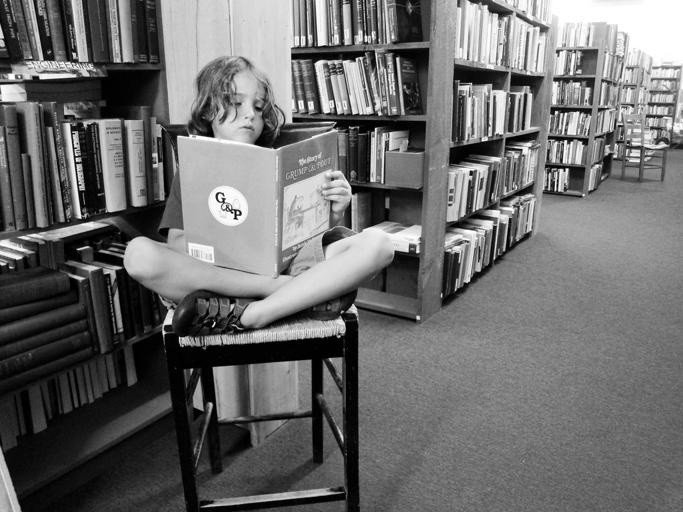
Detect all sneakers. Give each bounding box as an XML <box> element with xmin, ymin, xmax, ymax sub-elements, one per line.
<box><xmin>299</xmin><ymin>288</ymin><xmax>358</xmax><ymax>321</ymax></box>
<box><xmin>173</xmin><ymin>290</ymin><xmax>258</xmax><ymax>336</ymax></box>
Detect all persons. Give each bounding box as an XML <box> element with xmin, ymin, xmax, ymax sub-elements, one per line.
<box><xmin>120</xmin><ymin>53</ymin><xmax>396</xmax><ymax>340</ymax></box>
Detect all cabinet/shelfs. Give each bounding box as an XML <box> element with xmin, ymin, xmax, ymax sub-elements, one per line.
<box><xmin>294</xmin><ymin>1</ymin><xmax>558</xmax><ymax>325</ymax></box>
<box><xmin>545</xmin><ymin>21</ymin><xmax>683</xmax><ymax>197</ymax></box>
<box><xmin>0</xmin><ymin>0</ymin><xmax>295</xmax><ymax>512</ymax></box>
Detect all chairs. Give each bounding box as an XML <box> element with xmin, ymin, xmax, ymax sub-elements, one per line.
<box><xmin>159</xmin><ymin>121</ymin><xmax>360</xmax><ymax>512</ymax></box>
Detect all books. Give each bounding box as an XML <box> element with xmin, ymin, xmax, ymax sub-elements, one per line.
<box><xmin>175</xmin><ymin>128</ymin><xmax>338</xmax><ymax>279</ymax></box>
<box><xmin>540</xmin><ymin>21</ymin><xmax>682</xmax><ymax>195</ymax></box>
